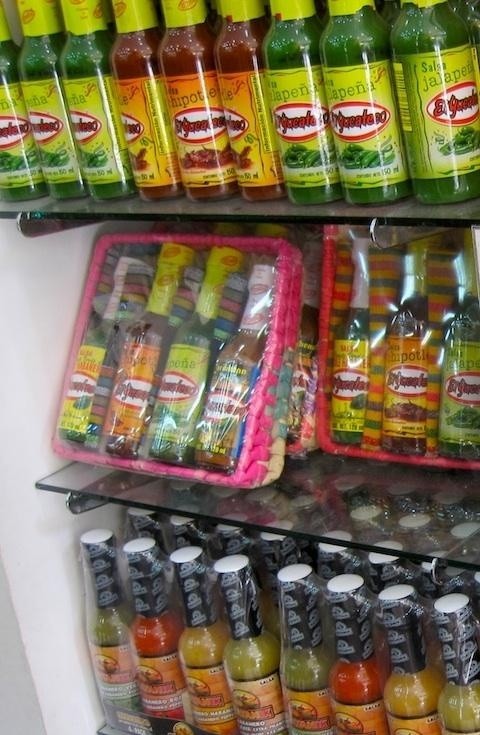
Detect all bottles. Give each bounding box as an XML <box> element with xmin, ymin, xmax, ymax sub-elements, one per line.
<box><xmin>143</xmin><ymin>248</ymin><xmax>243</xmax><ymax>466</ymax></box>
<box><xmin>126</xmin><ymin>460</ymin><xmax>478</xmax><ymax>591</ymax></box>
<box><xmin>330</xmin><ymin>238</ymin><xmax>370</xmax><ymax>447</ymax></box>
<box><xmin>320</xmin><ymin>0</ymin><xmax>413</xmax><ymax>205</ymax></box>
<box><xmin>55</xmin><ymin>0</ymin><xmax>139</xmax><ymax>201</ymax></box>
<box><xmin>111</xmin><ymin>0</ymin><xmax>186</xmax><ymax>200</ymax></box>
<box><xmin>214</xmin><ymin>555</ymin><xmax>284</xmax><ymax>735</ymax></box>
<box><xmin>193</xmin><ymin>257</ymin><xmax>275</xmax><ymax>471</ymax></box>
<box><xmin>0</xmin><ymin>2</ymin><xmax>48</xmax><ymax>201</ymax></box>
<box><xmin>169</xmin><ymin>547</ymin><xmax>239</xmax><ymax>735</ymax></box>
<box><xmin>57</xmin><ymin>243</ymin><xmax>121</xmax><ymax>444</ymax></box>
<box><xmin>434</xmin><ymin>593</ymin><xmax>480</xmax><ymax>735</ymax></box>
<box><xmin>438</xmin><ymin>228</ymin><xmax>480</xmax><ymax>459</ymax></box>
<box><xmin>97</xmin><ymin>243</ymin><xmax>198</xmax><ymax>460</ymax></box>
<box><xmin>123</xmin><ymin>537</ymin><xmax>187</xmax><ymax>720</ymax></box>
<box><xmin>326</xmin><ymin>575</ymin><xmax>391</xmax><ymax>734</ymax></box>
<box><xmin>214</xmin><ymin>0</ymin><xmax>291</xmax><ymax>201</ymax></box>
<box><xmin>380</xmin><ymin>233</ymin><xmax>428</xmax><ymax>454</ymax></box>
<box><xmin>158</xmin><ymin>0</ymin><xmax>239</xmax><ymax>201</ymax></box>
<box><xmin>378</xmin><ymin>585</ymin><xmax>445</xmax><ymax>735</ymax></box>
<box><xmin>81</xmin><ymin>529</ymin><xmax>138</xmax><ymax>713</ymax></box>
<box><xmin>277</xmin><ymin>565</ymin><xmax>335</xmax><ymax>734</ymax></box>
<box><xmin>260</xmin><ymin>0</ymin><xmax>344</xmax><ymax>205</ymax></box>
<box><xmin>389</xmin><ymin>0</ymin><xmax>480</xmax><ymax>204</ymax></box>
<box><xmin>15</xmin><ymin>0</ymin><xmax>89</xmax><ymax>199</ymax></box>
